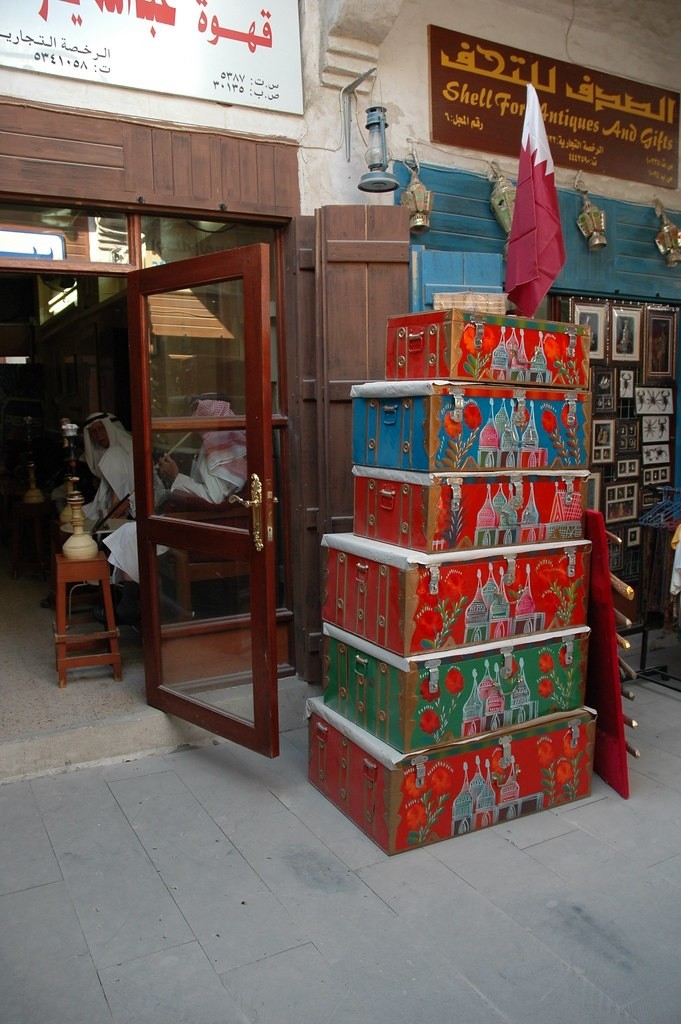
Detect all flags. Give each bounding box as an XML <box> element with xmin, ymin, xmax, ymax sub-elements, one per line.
<box><xmin>504</xmin><ymin>83</ymin><xmax>566</xmax><ymax>318</ymax></box>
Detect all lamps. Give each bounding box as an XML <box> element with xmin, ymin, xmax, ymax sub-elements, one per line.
<box><xmin>643</xmin><ymin>198</ymin><xmax>681</xmax><ymax>269</ymax></box>
<box><xmin>574</xmin><ymin>173</ymin><xmax>610</xmax><ymax>251</ymax></box>
<box><xmin>334</xmin><ymin>69</ymin><xmax>394</xmax><ymax>192</ymax></box>
<box><xmin>400</xmin><ymin>154</ymin><xmax>436</xmax><ymax>237</ymax></box>
<box><xmin>481</xmin><ymin>161</ymin><xmax>516</xmax><ymax>258</ymax></box>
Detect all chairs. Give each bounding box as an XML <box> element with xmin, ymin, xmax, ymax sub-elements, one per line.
<box><xmin>168</xmin><ymin>508</ymin><xmax>264</xmax><ymax>620</ymax></box>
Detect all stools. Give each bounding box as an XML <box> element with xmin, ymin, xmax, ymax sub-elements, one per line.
<box><xmin>53</xmin><ymin>553</ymin><xmax>123</xmax><ymax>688</ymax></box>
<box><xmin>10</xmin><ymin>498</ymin><xmax>54</xmax><ymax>584</ymax></box>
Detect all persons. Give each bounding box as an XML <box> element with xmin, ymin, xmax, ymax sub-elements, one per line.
<box><xmin>41</xmin><ymin>398</ymin><xmax>247</xmax><ymax>626</ymax></box>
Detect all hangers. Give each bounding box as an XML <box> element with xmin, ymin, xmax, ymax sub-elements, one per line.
<box><xmin>637</xmin><ymin>486</ymin><xmax>681</xmax><ymax>528</ymax></box>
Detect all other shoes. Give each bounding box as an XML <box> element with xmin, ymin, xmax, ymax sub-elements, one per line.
<box><xmin>40</xmin><ymin>596</ymin><xmax>52</xmax><ymax>608</ymax></box>
<box><xmin>92</xmin><ymin>607</ymin><xmax>141</xmax><ymax>625</ymax></box>
<box><xmin>132</xmin><ymin>623</ymin><xmax>175</xmax><ymax>635</ymax></box>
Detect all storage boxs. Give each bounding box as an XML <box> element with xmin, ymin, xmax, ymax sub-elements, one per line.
<box><xmin>317</xmin><ymin>623</ymin><xmax>596</xmax><ymax>752</ymax></box>
<box><xmin>318</xmin><ymin>533</ymin><xmax>599</xmax><ymax>655</ymax></box>
<box><xmin>351</xmin><ymin>385</ymin><xmax>599</xmax><ymax>479</ymax></box>
<box><xmin>383</xmin><ymin>307</ymin><xmax>608</xmax><ymax>388</ymax></box>
<box><xmin>296</xmin><ymin>696</ymin><xmax>615</xmax><ymax>858</ymax></box>
<box><xmin>354</xmin><ymin>467</ymin><xmax>593</xmax><ymax>554</ymax></box>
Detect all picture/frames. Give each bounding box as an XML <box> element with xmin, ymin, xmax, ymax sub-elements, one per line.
<box><xmin>589</xmin><ymin>416</ymin><xmax>617</xmax><ymax>463</ymax></box>
<box><xmin>590</xmin><ymin>366</ymin><xmax>617</xmax><ymax>412</ymax></box>
<box><xmin>616</xmin><ymin>369</ymin><xmax>636</xmax><ymax>399</ymax></box>
<box><xmin>624</xmin><ymin>526</ymin><xmax>642</xmax><ymax>548</ymax></box>
<box><xmin>610</xmin><ymin>302</ymin><xmax>646</xmax><ymax>364</ymax></box>
<box><xmin>641</xmin><ymin>415</ymin><xmax>672</xmax><ymax>443</ymax></box>
<box><xmin>605</xmin><ymin>484</ymin><xmax>639</xmax><ymax>526</ymax></box>
<box><xmin>641</xmin><ymin>305</ymin><xmax>679</xmax><ymax>387</ymax></box>
<box><xmin>615</xmin><ymin>417</ymin><xmax>641</xmax><ymax>459</ymax></box>
<box><xmin>635</xmin><ymin>385</ymin><xmax>674</xmax><ymax>415</ymax></box>
<box><xmin>639</xmin><ymin>442</ymin><xmax>673</xmax><ymax>465</ymax></box>
<box><xmin>567</xmin><ymin>292</ymin><xmax>609</xmax><ymax>361</ymax></box>
<box><xmin>643</xmin><ymin>464</ymin><xmax>671</xmax><ymax>485</ymax></box>
<box><xmin>614</xmin><ymin>454</ymin><xmax>643</xmax><ymax>484</ymax></box>
<box><xmin>639</xmin><ymin>490</ymin><xmax>676</xmax><ymax>511</ymax></box>
